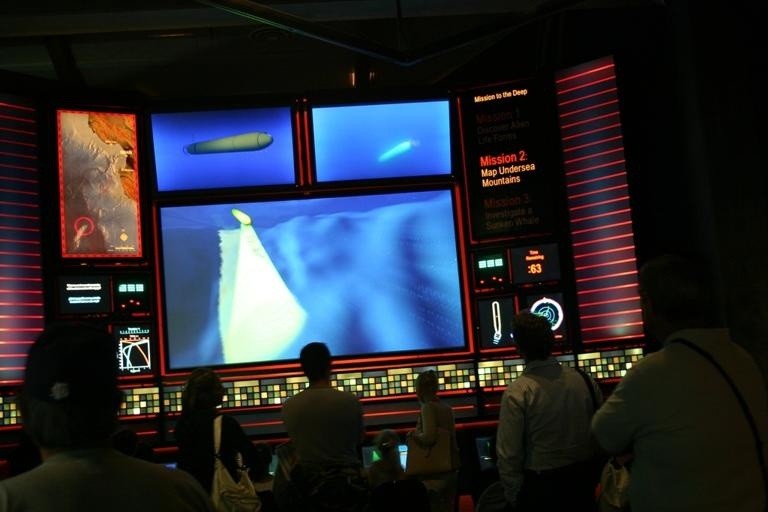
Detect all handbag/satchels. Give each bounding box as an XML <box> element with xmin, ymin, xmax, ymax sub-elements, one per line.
<box><xmin>405</xmin><ymin>436</ymin><xmax>452</xmax><ymax>478</ymax></box>
<box><xmin>212</xmin><ymin>460</ymin><xmax>261</xmax><ymax>511</ymax></box>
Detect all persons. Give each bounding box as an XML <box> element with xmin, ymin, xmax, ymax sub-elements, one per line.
<box><xmin>1</xmin><ymin>316</ymin><xmax>458</xmax><ymax>512</ymax></box>
<box><xmin>496</xmin><ymin>311</ymin><xmax>605</xmax><ymax>512</ymax></box>
<box><xmin>593</xmin><ymin>255</ymin><xmax>766</xmax><ymax>512</ymax></box>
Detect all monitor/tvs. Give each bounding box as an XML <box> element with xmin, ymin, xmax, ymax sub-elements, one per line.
<box><xmin>149</xmin><ymin>185</ymin><xmax>478</xmax><ymax>380</ymax></box>
<box><xmin>301</xmin><ymin>93</ymin><xmax>466</xmax><ymax>191</ymax></box>
<box><xmin>143</xmin><ymin>98</ymin><xmax>304</xmax><ymax>197</ymax></box>
